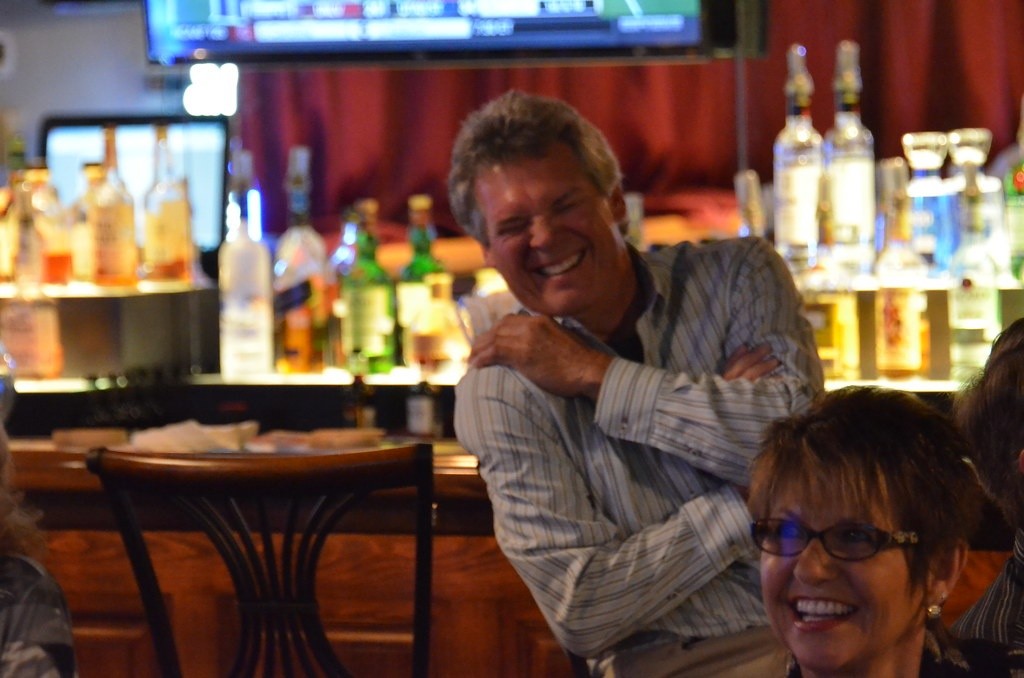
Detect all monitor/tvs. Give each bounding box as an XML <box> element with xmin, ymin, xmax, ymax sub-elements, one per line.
<box><xmin>139</xmin><ymin>0</ymin><xmax>767</xmax><ymax>60</ymax></box>
<box><xmin>41</xmin><ymin>114</ymin><xmax>230</xmax><ymax>275</ymax></box>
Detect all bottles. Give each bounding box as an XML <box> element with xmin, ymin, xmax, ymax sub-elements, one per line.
<box><xmin>774</xmin><ymin>34</ymin><xmax>1024</xmax><ymax>386</ymax></box>
<box><xmin>0</xmin><ymin>117</ymin><xmax>474</xmax><ymax>379</ymax></box>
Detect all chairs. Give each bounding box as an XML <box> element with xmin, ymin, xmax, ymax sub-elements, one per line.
<box><xmin>83</xmin><ymin>444</ymin><xmax>438</xmax><ymax>678</ymax></box>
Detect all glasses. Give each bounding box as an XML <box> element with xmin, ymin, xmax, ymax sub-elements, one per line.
<box><xmin>750</xmin><ymin>517</ymin><xmax>920</xmax><ymax>562</ymax></box>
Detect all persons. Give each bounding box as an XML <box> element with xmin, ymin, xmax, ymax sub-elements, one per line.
<box><xmin>745</xmin><ymin>386</ymin><xmax>1024</xmax><ymax>678</ymax></box>
<box><xmin>954</xmin><ymin>316</ymin><xmax>1024</xmax><ymax>644</ymax></box>
<box><xmin>448</xmin><ymin>90</ymin><xmax>823</xmax><ymax>678</ymax></box>
